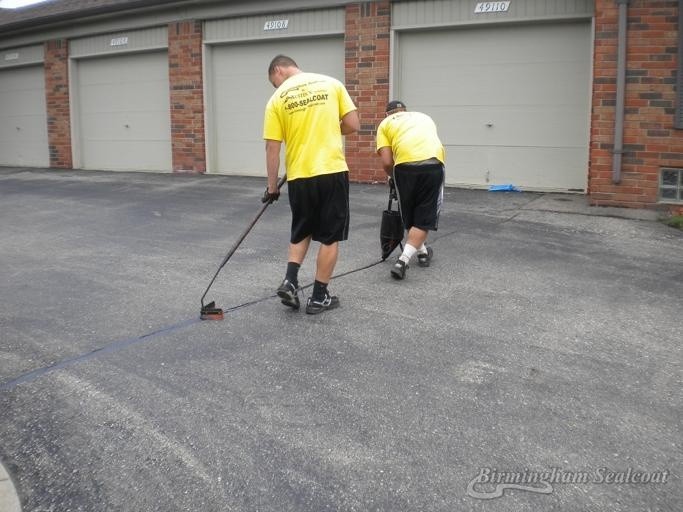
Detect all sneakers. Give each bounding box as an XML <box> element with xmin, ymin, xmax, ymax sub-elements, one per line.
<box><xmin>276</xmin><ymin>278</ymin><xmax>300</xmax><ymax>309</ymax></box>
<box><xmin>417</xmin><ymin>246</ymin><xmax>433</xmax><ymax>268</ymax></box>
<box><xmin>391</xmin><ymin>260</ymin><xmax>409</xmax><ymax>279</ymax></box>
<box><xmin>306</xmin><ymin>296</ymin><xmax>340</xmax><ymax>315</ymax></box>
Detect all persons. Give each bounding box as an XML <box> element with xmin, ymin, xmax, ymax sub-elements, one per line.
<box><xmin>263</xmin><ymin>54</ymin><xmax>360</xmax><ymax>314</ymax></box>
<box><xmin>376</xmin><ymin>101</ymin><xmax>447</xmax><ymax>279</ymax></box>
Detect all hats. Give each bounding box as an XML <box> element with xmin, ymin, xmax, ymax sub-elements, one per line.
<box><xmin>385</xmin><ymin>100</ymin><xmax>407</xmax><ymax>111</ymax></box>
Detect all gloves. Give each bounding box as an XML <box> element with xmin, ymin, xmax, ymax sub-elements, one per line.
<box><xmin>261</xmin><ymin>187</ymin><xmax>281</xmax><ymax>204</ymax></box>
